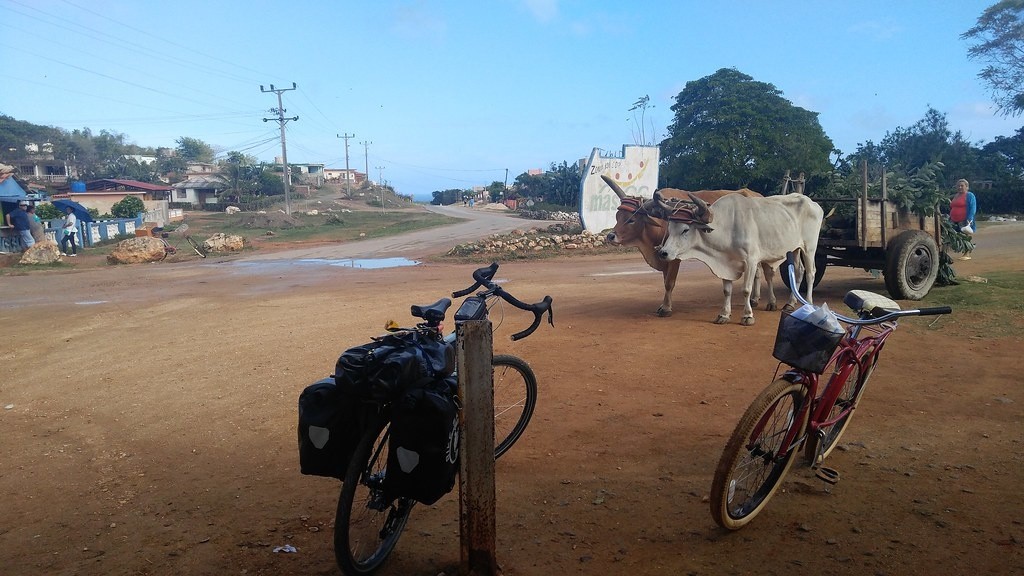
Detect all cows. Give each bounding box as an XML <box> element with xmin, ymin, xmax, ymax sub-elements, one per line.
<box><xmin>600</xmin><ymin>174</ymin><xmax>777</xmax><ymax>317</ymax></box>
<box><xmin>653</xmin><ymin>189</ymin><xmax>824</xmax><ymax>326</ymax></box>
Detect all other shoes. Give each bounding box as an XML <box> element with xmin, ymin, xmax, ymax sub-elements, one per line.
<box><xmin>61</xmin><ymin>253</ymin><xmax>67</xmax><ymax>256</ymax></box>
<box><xmin>68</xmin><ymin>254</ymin><xmax>76</xmax><ymax>257</ymax></box>
<box><xmin>957</xmin><ymin>257</ymin><xmax>971</xmax><ymax>260</ymax></box>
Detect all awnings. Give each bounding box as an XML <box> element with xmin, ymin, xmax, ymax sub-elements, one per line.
<box><xmin>0</xmin><ymin>197</ymin><xmax>41</xmax><ymax>202</ymax></box>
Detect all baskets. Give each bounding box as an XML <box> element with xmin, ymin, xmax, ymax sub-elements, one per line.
<box><xmin>175</xmin><ymin>224</ymin><xmax>189</xmax><ymax>235</ymax></box>
<box><xmin>772</xmin><ymin>303</ymin><xmax>846</xmax><ymax>375</ymax></box>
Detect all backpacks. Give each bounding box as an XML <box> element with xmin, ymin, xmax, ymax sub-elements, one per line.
<box><xmin>334</xmin><ymin>329</ymin><xmax>456</xmax><ymax>399</ymax></box>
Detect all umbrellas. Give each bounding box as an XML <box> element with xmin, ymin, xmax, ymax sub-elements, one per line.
<box><xmin>52</xmin><ymin>200</ymin><xmax>93</xmax><ymax>223</ymax></box>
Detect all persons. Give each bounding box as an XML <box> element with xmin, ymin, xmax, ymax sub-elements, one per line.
<box><xmin>463</xmin><ymin>195</ymin><xmax>468</xmax><ymax>207</ymax></box>
<box><xmin>950</xmin><ymin>179</ymin><xmax>977</xmax><ymax>260</ymax></box>
<box><xmin>61</xmin><ymin>206</ymin><xmax>78</xmax><ymax>257</ymax></box>
<box><xmin>6</xmin><ymin>201</ymin><xmax>44</xmax><ymax>252</ymax></box>
<box><xmin>470</xmin><ymin>198</ymin><xmax>474</xmax><ymax>206</ymax></box>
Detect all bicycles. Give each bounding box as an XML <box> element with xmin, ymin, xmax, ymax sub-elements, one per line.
<box><xmin>152</xmin><ymin>216</ymin><xmax>207</xmax><ymax>262</ymax></box>
<box><xmin>333</xmin><ymin>262</ymin><xmax>553</xmax><ymax>575</ymax></box>
<box><xmin>711</xmin><ymin>251</ymin><xmax>952</xmax><ymax>531</ymax></box>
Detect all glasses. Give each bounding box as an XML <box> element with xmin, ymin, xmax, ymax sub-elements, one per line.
<box><xmin>20</xmin><ymin>204</ymin><xmax>28</xmax><ymax>206</ymax></box>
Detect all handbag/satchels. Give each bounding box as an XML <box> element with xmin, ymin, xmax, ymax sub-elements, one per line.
<box><xmin>961</xmin><ymin>220</ymin><xmax>974</xmax><ymax>236</ymax></box>
